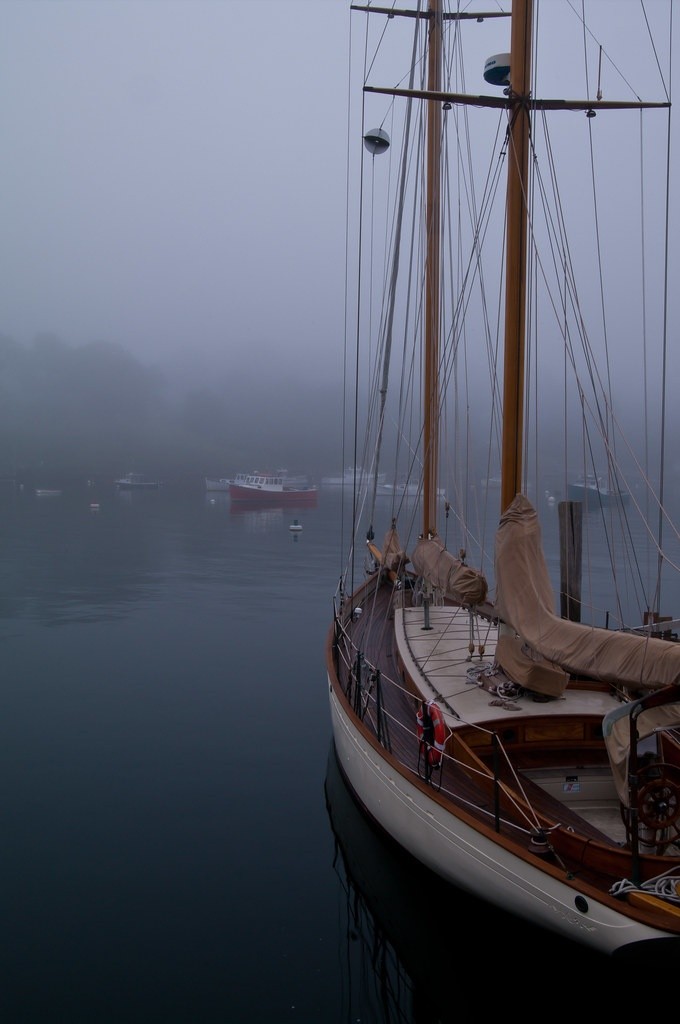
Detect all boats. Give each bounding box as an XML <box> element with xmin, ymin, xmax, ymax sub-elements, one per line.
<box><xmin>112</xmin><ymin>472</ymin><xmax>160</xmax><ymax>490</ymax></box>
<box><xmin>321</xmin><ymin>467</ymin><xmax>386</xmax><ymax>485</ymax></box>
<box><xmin>480</xmin><ymin>470</ymin><xmax>532</xmax><ymax>489</ymax></box>
<box><xmin>290</xmin><ymin>524</ymin><xmax>303</xmax><ymax>531</ymax></box>
<box><xmin>31</xmin><ymin>488</ymin><xmax>62</xmax><ymax>493</ymax></box>
<box><xmin>231</xmin><ymin>501</ymin><xmax>318</xmax><ymax>513</ymax></box>
<box><xmin>205</xmin><ymin>470</ymin><xmax>284</xmax><ymax>492</ymax></box>
<box><xmin>375</xmin><ymin>478</ymin><xmax>447</xmax><ymax>497</ymax></box>
<box><xmin>229</xmin><ymin>483</ymin><xmax>318</xmax><ymax>500</ymax></box>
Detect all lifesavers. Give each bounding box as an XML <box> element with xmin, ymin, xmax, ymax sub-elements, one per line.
<box><xmin>416</xmin><ymin>701</ymin><xmax>446</xmax><ymax>770</ymax></box>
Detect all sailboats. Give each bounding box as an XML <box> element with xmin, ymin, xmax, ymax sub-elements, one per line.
<box><xmin>322</xmin><ymin>728</ymin><xmax>680</xmax><ymax>1024</ymax></box>
<box><xmin>326</xmin><ymin>0</ymin><xmax>680</xmax><ymax>1012</ymax></box>
